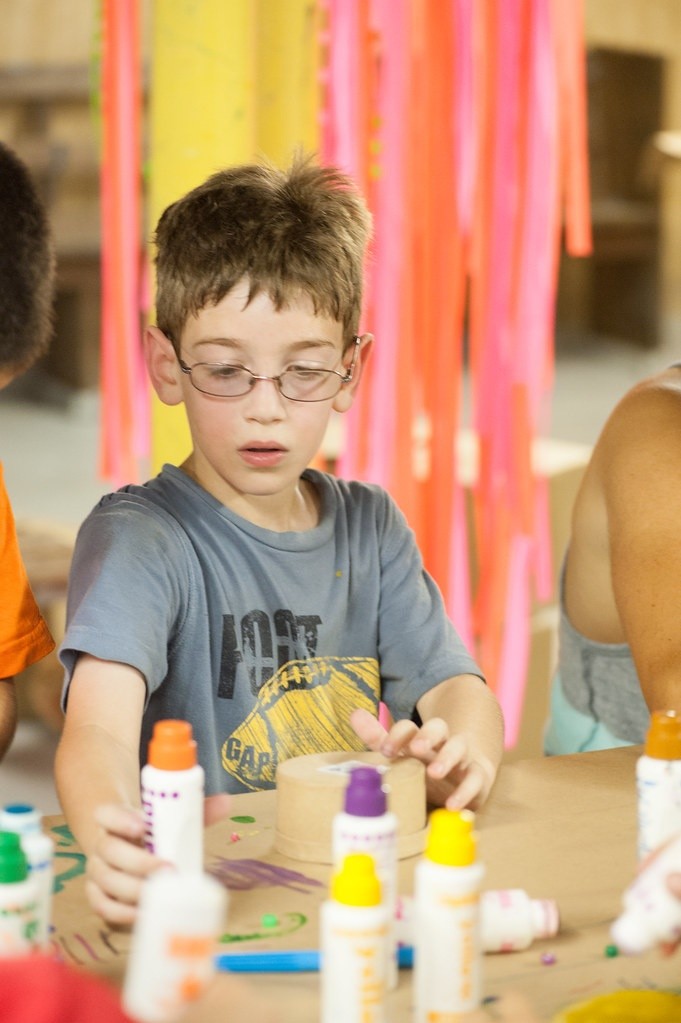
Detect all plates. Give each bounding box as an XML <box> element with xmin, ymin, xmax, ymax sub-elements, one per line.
<box><xmin>549</xmin><ymin>990</ymin><xmax>681</xmax><ymax>1023</ymax></box>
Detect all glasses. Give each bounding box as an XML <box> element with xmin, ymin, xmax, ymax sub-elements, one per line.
<box><xmin>165</xmin><ymin>333</ymin><xmax>361</xmax><ymax>401</ymax></box>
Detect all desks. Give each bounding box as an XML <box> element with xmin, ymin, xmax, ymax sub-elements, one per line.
<box><xmin>42</xmin><ymin>745</ymin><xmax>680</xmax><ymax>1022</ymax></box>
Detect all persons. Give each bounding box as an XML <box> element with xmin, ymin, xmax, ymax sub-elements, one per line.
<box><xmin>539</xmin><ymin>356</ymin><xmax>681</xmax><ymax>755</ymax></box>
<box><xmin>0</xmin><ymin>132</ymin><xmax>56</xmax><ymax>762</ymax></box>
<box><xmin>49</xmin><ymin>156</ymin><xmax>506</xmax><ymax>933</ymax></box>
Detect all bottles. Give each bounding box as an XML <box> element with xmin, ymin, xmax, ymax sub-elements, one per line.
<box><xmin>610</xmin><ymin>709</ymin><xmax>681</xmax><ymax>955</ymax></box>
<box><xmin>410</xmin><ymin>807</ymin><xmax>485</xmax><ymax>1023</ymax></box>
<box><xmin>320</xmin><ymin>766</ymin><xmax>398</xmax><ymax>1023</ymax></box>
<box><xmin>400</xmin><ymin>888</ymin><xmax>561</xmax><ymax>952</ymax></box>
<box><xmin>0</xmin><ymin>800</ymin><xmax>56</xmax><ymax>952</ymax></box>
<box><xmin>120</xmin><ymin>716</ymin><xmax>230</xmax><ymax>1023</ymax></box>
